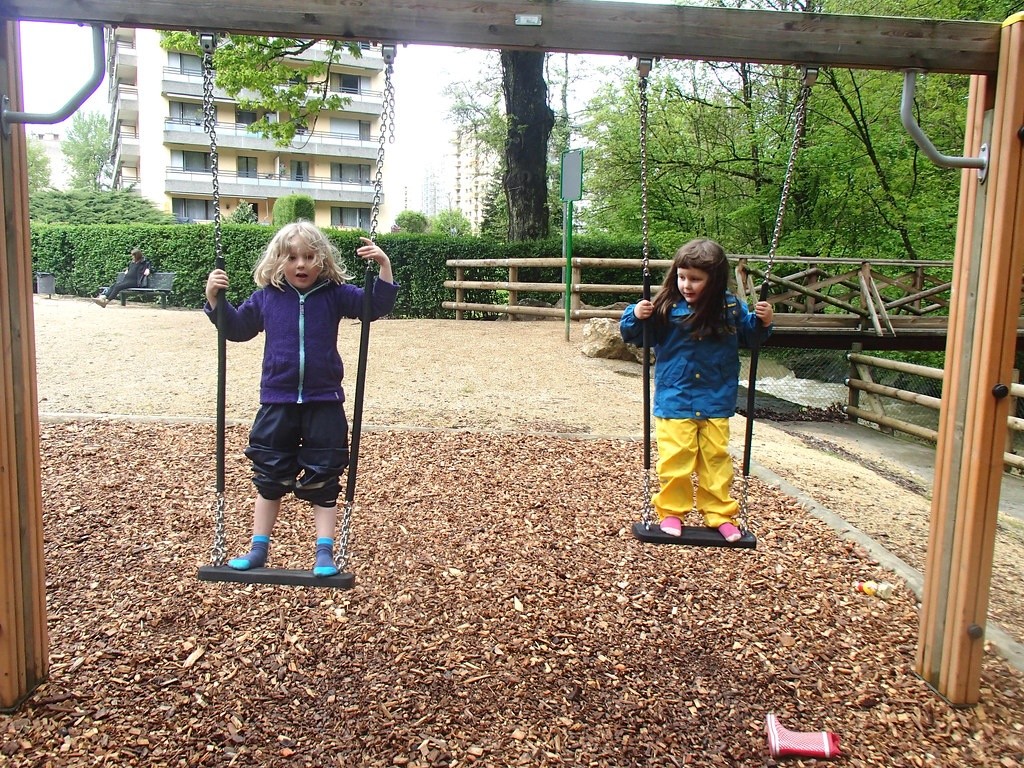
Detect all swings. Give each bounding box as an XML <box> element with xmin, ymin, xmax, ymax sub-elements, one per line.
<box><xmin>630</xmin><ymin>77</ymin><xmax>816</xmax><ymax>547</ymax></box>
<box><xmin>197</xmin><ymin>38</ymin><xmax>399</xmax><ymax>588</ymax></box>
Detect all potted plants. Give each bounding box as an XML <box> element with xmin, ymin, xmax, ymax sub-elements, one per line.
<box><xmin>194</xmin><ymin>108</ymin><xmax>202</xmax><ymax>125</ymax></box>
<box><xmin>280</xmin><ymin>167</ymin><xmax>288</xmax><ymax>180</ymax></box>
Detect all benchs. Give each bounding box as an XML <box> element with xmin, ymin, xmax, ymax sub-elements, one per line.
<box><xmin>99</xmin><ymin>272</ymin><xmax>177</xmax><ymax>309</ymax></box>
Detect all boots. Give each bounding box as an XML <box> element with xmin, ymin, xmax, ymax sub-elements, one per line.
<box><xmin>767</xmin><ymin>713</ymin><xmax>843</xmax><ymax>758</ymax></box>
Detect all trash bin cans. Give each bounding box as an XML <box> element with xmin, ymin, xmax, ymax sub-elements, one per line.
<box><xmin>35</xmin><ymin>271</ymin><xmax>56</xmax><ymax>294</ymax></box>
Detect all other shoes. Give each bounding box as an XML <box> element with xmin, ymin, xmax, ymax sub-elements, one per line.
<box><xmin>92</xmin><ymin>295</ymin><xmax>107</xmax><ymax>306</ymax></box>
<box><xmin>101</xmin><ymin>299</ymin><xmax>110</xmax><ymax>308</ymax></box>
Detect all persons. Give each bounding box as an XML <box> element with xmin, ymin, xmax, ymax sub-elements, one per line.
<box><xmin>91</xmin><ymin>248</ymin><xmax>156</xmax><ymax>308</ymax></box>
<box><xmin>204</xmin><ymin>222</ymin><xmax>401</xmax><ymax>577</ymax></box>
<box><xmin>621</xmin><ymin>236</ymin><xmax>773</xmax><ymax>542</ymax></box>
